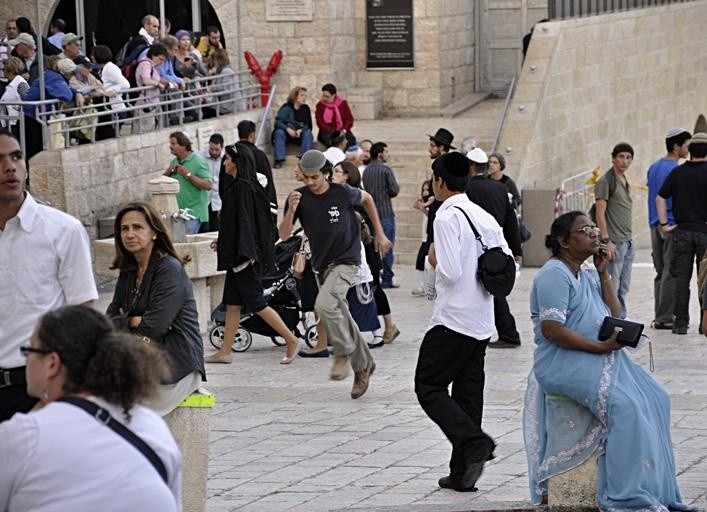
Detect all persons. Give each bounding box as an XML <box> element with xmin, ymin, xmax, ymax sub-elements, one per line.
<box><xmin>2</xmin><ymin>12</ymin><xmax>247</xmax><ymax>154</ymax></box>
<box><xmin>1</xmin><ymin>129</ymin><xmax>99</xmax><ymax>422</ymax></box>
<box><xmin>415</xmin><ymin>128</ymin><xmax>529</xmax><ymax>348</ymax></box>
<box><xmin>531</xmin><ymin>211</ymin><xmax>675</xmax><ymax>507</ymax></box>
<box><xmin>2</xmin><ymin>306</ymin><xmax>180</xmax><ymax>512</ymax></box>
<box><xmin>109</xmin><ymin>202</ymin><xmax>206</xmax><ymax>417</ymax></box>
<box><xmin>161</xmin><ymin>82</ymin><xmax>399</xmax><ymax>399</ymax></box>
<box><xmin>656</xmin><ymin>132</ymin><xmax>706</xmax><ymax>334</ymax></box>
<box><xmin>594</xmin><ymin>143</ymin><xmax>634</xmax><ymax>320</ymax></box>
<box><xmin>648</xmin><ymin>128</ymin><xmax>692</xmax><ymax>328</ymax></box>
<box><xmin>413</xmin><ymin>154</ymin><xmax>512</xmax><ymax>490</ymax></box>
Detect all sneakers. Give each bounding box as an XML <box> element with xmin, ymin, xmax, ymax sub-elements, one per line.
<box><xmin>489</xmin><ymin>339</ymin><xmax>517</xmax><ymax>348</ymax></box>
<box><xmin>412</xmin><ymin>287</ymin><xmax>426</xmax><ymax>296</ymax></box>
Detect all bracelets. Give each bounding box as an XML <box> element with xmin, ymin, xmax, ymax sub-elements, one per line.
<box><xmin>601</xmin><ymin>275</ymin><xmax>613</xmax><ymax>286</ymax></box>
<box><xmin>661</xmin><ymin>220</ymin><xmax>668</xmax><ymax>227</ymax></box>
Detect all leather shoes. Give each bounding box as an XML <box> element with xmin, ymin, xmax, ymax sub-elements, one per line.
<box><xmin>367</xmin><ymin>324</ymin><xmax>399</xmax><ymax>348</ymax></box>
<box><xmin>439</xmin><ymin>439</ymin><xmax>493</xmax><ymax>492</ymax></box>
<box><xmin>274</xmin><ymin>161</ymin><xmax>281</xmax><ymax>168</ymax></box>
<box><xmin>205</xmin><ymin>356</ymin><xmax>231</xmax><ymax>362</ymax></box>
<box><xmin>654</xmin><ymin>321</ymin><xmax>687</xmax><ymax>334</ymax></box>
<box><xmin>351</xmin><ymin>359</ymin><xmax>374</xmax><ymax>398</ymax></box>
<box><xmin>381</xmin><ymin>283</ymin><xmax>399</xmax><ymax>288</ymax></box>
<box><xmin>280</xmin><ymin>340</ymin><xmax>350</xmax><ymax>381</ymax></box>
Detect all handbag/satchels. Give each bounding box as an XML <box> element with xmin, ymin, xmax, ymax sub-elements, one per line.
<box><xmin>598</xmin><ymin>317</ymin><xmax>643</xmax><ymax>347</ymax></box>
<box><xmin>478</xmin><ymin>247</ymin><xmax>515</xmax><ymax>296</ymax></box>
<box><xmin>131</xmin><ymin>107</ymin><xmax>155</xmax><ymax>133</ymax></box>
<box><xmin>519</xmin><ymin>224</ymin><xmax>530</xmax><ymax>242</ymax></box>
<box><xmin>70</xmin><ymin>108</ymin><xmax>98</xmax><ymax>142</ymax></box>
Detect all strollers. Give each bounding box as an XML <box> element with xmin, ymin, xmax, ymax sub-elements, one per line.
<box><xmin>208</xmin><ymin>227</ymin><xmax>320</xmax><ymax>352</ymax></box>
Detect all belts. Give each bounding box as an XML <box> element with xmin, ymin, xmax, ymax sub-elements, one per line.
<box><xmin>0</xmin><ymin>369</ymin><xmax>26</xmax><ymax>388</ymax></box>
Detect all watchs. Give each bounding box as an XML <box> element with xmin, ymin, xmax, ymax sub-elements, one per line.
<box><xmin>598</xmin><ymin>236</ymin><xmax>610</xmax><ymax>247</ymax></box>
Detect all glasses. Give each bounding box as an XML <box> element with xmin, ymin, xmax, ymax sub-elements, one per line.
<box><xmin>21</xmin><ymin>345</ymin><xmax>48</xmax><ymax>357</ymax></box>
<box><xmin>570</xmin><ymin>226</ymin><xmax>599</xmax><ymax>234</ymax></box>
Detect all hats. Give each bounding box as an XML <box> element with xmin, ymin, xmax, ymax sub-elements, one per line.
<box><xmin>466</xmin><ymin>148</ymin><xmax>505</xmax><ymax>166</ymax></box>
<box><xmin>73</xmin><ymin>55</ymin><xmax>94</xmax><ymax>66</ymax></box>
<box><xmin>62</xmin><ymin>33</ymin><xmax>83</xmax><ymax>46</ymax></box>
<box><xmin>9</xmin><ymin>32</ymin><xmax>35</xmax><ymax>46</ymax></box>
<box><xmin>427</xmin><ymin>128</ymin><xmax>457</xmax><ymax>149</ymax></box>
<box><xmin>300</xmin><ymin>150</ymin><xmax>326</xmax><ymax>171</ymax></box>
<box><xmin>176</xmin><ymin>30</ymin><xmax>188</xmax><ymax>39</ymax></box>
<box><xmin>666</xmin><ymin>127</ymin><xmax>707</xmax><ymax>143</ymax></box>
